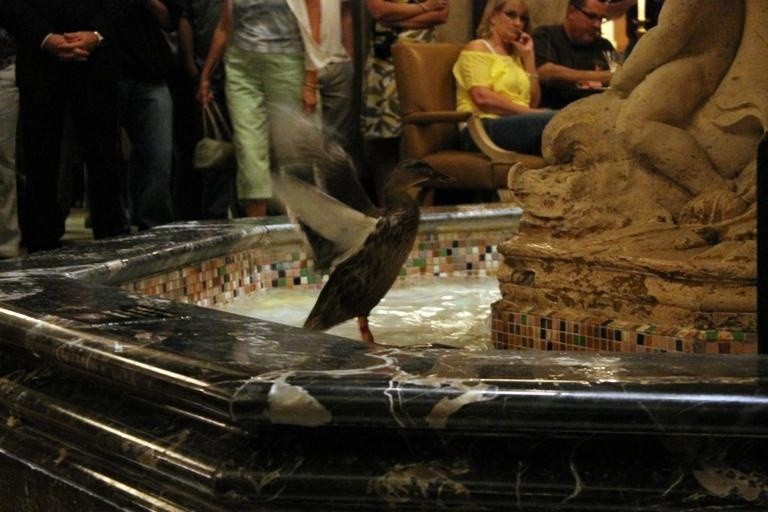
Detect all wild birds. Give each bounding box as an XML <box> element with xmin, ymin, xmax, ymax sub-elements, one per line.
<box><xmin>267</xmin><ymin>128</ymin><xmax>458</xmax><ymax>343</ymax></box>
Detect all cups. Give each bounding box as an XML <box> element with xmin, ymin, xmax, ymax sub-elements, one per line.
<box><xmin>602</xmin><ymin>48</ymin><xmax>627</xmax><ymax>73</ymax></box>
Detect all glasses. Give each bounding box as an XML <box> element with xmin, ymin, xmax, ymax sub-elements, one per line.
<box><xmin>569</xmin><ymin>2</ymin><xmax>607</xmax><ymax>22</ymax></box>
<box><xmin>504</xmin><ymin>9</ymin><xmax>529</xmax><ymax>22</ymax></box>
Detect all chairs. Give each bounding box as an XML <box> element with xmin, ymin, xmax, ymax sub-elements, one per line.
<box><xmin>386</xmin><ymin>36</ymin><xmax>543</xmax><ymax>207</ymax></box>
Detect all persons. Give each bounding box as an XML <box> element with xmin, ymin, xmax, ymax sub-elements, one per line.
<box><xmin>610</xmin><ymin>0</ymin><xmax>745</xmax><ymax>194</ymax></box>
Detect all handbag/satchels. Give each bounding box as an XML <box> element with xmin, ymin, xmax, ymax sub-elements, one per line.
<box><xmin>194</xmin><ymin>137</ymin><xmax>234</xmax><ymax>170</ymax></box>
